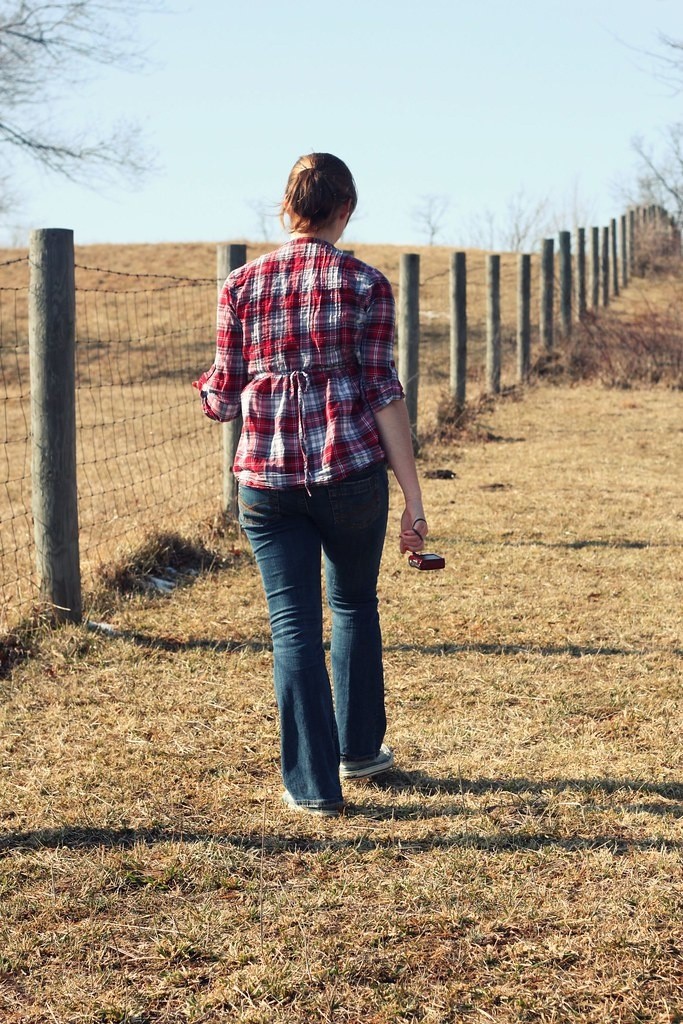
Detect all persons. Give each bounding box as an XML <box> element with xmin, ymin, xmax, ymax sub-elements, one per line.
<box><xmin>195</xmin><ymin>153</ymin><xmax>428</xmax><ymax>818</ymax></box>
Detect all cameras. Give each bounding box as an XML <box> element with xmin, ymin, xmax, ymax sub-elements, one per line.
<box><xmin>408</xmin><ymin>553</ymin><xmax>445</xmax><ymax>570</ymax></box>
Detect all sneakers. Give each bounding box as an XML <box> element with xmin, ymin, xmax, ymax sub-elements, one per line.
<box><xmin>282</xmin><ymin>790</ymin><xmax>339</xmax><ymax>818</ymax></box>
<box><xmin>338</xmin><ymin>742</ymin><xmax>393</xmax><ymax>781</ymax></box>
